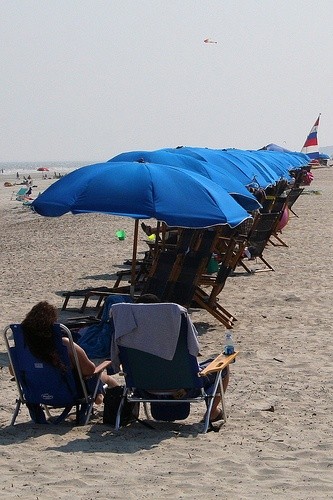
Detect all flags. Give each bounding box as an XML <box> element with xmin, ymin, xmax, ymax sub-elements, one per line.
<box><xmin>304</xmin><ymin>115</ymin><xmax>319</xmax><ymax>147</ymax></box>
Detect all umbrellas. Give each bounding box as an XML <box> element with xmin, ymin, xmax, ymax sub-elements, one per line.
<box><xmin>307</xmin><ymin>152</ymin><xmax>330</xmax><ymax>159</ymax></box>
<box><xmin>30</xmin><ymin>144</ymin><xmax>311</xmax><ymax>186</ymax></box>
<box><xmin>31</xmin><ymin>160</ymin><xmax>252</xmax><ymax>303</ymax></box>
<box><xmin>100</xmin><ymin>150</ymin><xmax>265</xmax><ymax>268</ymax></box>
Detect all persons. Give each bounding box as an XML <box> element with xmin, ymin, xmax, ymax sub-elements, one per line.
<box><xmin>118</xmin><ymin>295</ymin><xmax>230</xmax><ymax>421</ymax></box>
<box><xmin>141</xmin><ymin>221</ymin><xmax>174</xmax><ymax>235</ymax></box>
<box><xmin>20</xmin><ymin>301</ymin><xmax>118</xmax><ymax>405</ymax></box>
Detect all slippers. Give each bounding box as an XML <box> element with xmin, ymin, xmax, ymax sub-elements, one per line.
<box><xmin>202</xmin><ymin>407</ymin><xmax>228</xmax><ymax>422</ymax></box>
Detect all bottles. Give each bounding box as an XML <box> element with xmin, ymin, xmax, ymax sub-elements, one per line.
<box><xmin>222</xmin><ymin>331</ymin><xmax>235</xmax><ymax>356</ymax></box>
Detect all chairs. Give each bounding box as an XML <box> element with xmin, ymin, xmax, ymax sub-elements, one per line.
<box><xmin>113</xmin><ymin>304</ymin><xmax>228</xmax><ymax>435</ymax></box>
<box><xmin>62</xmin><ymin>221</ymin><xmax>252</xmax><ymax>326</ymax></box>
<box><xmin>251</xmin><ymin>164</ymin><xmax>310</xmax><ymax>270</ymax></box>
<box><xmin>2</xmin><ymin>324</ymin><xmax>110</xmax><ymax>430</ymax></box>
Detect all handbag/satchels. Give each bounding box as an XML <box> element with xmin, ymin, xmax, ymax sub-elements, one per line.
<box><xmin>103</xmin><ymin>385</ymin><xmax>139</xmax><ymax>426</ymax></box>
